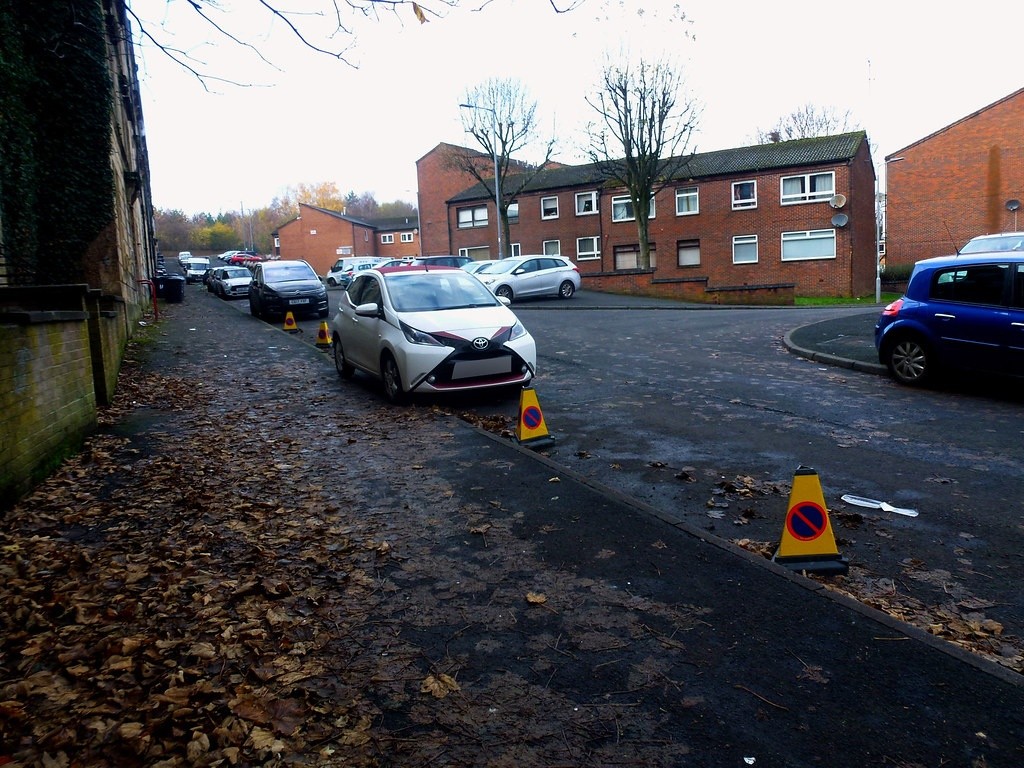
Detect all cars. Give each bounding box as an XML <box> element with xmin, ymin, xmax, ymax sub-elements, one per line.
<box><xmin>204</xmin><ymin>266</ymin><xmax>254</xmax><ymax>300</ymax></box>
<box><xmin>340</xmin><ymin>264</ymin><xmax>379</xmax><ymax>287</ymax></box>
<box><xmin>952</xmin><ymin>232</ymin><xmax>1024</xmax><ymax>282</ymax></box>
<box><xmin>334</xmin><ymin>265</ymin><xmax>538</xmax><ymax>402</ymax></box>
<box><xmin>218</xmin><ymin>250</ymin><xmax>237</xmax><ymax>260</ymax></box>
<box><xmin>411</xmin><ymin>257</ymin><xmax>474</xmax><ymax>269</ymax></box>
<box><xmin>440</xmin><ymin>260</ymin><xmax>502</xmax><ymax>290</ymax></box>
<box><xmin>178</xmin><ymin>252</ymin><xmax>193</xmax><ymax>266</ymax></box>
<box><xmin>873</xmin><ymin>252</ymin><xmax>1024</xmax><ymax>387</ymax></box>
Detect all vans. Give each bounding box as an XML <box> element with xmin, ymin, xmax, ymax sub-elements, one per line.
<box><xmin>186</xmin><ymin>257</ymin><xmax>210</xmax><ymax>285</ymax></box>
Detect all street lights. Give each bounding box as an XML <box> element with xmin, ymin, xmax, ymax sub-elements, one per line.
<box><xmin>877</xmin><ymin>157</ymin><xmax>906</xmax><ymax>309</ymax></box>
<box><xmin>405</xmin><ymin>189</ymin><xmax>422</xmax><ymax>258</ymax></box>
<box><xmin>459</xmin><ymin>104</ymin><xmax>503</xmax><ymax>260</ymax></box>
<box><xmin>246</xmin><ymin>209</ymin><xmax>253</xmax><ymax>251</ymax></box>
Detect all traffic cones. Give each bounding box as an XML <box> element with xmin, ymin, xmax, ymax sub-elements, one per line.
<box><xmin>778</xmin><ymin>466</ymin><xmax>846</xmax><ymax>573</ymax></box>
<box><xmin>317</xmin><ymin>319</ymin><xmax>334</xmax><ymax>348</ymax></box>
<box><xmin>284</xmin><ymin>310</ymin><xmax>300</xmax><ymax>333</ymax></box>
<box><xmin>519</xmin><ymin>389</ymin><xmax>558</xmax><ymax>454</ymax></box>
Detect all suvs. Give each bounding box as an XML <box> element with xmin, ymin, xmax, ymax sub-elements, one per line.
<box><xmin>249</xmin><ymin>260</ymin><xmax>331</xmax><ymax>322</ymax></box>
<box><xmin>473</xmin><ymin>254</ymin><xmax>581</xmax><ymax>303</ymax></box>
<box><xmin>328</xmin><ymin>257</ymin><xmax>393</xmax><ymax>285</ymax></box>
<box><xmin>232</xmin><ymin>255</ymin><xmax>262</xmax><ymax>266</ymax></box>
<box><xmin>225</xmin><ymin>251</ymin><xmax>257</xmax><ymax>264</ymax></box>
